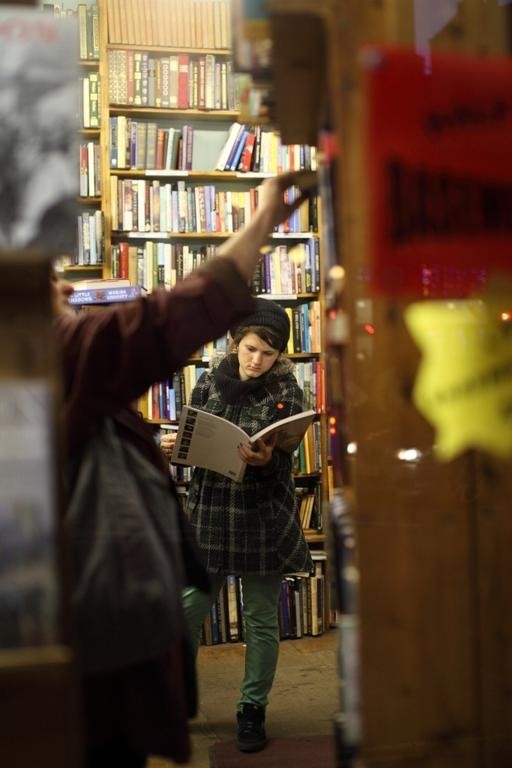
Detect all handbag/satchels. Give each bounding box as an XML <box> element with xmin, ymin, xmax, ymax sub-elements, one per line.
<box><xmin>52</xmin><ymin>436</ymin><xmax>178</xmax><ymax>675</ymax></box>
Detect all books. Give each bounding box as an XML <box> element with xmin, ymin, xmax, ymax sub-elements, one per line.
<box><xmin>46</xmin><ymin>0</ymin><xmax>334</xmax><ymax>648</ymax></box>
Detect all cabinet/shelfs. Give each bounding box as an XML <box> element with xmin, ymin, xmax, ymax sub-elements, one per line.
<box><xmin>58</xmin><ymin>1</ymin><xmax>336</xmax><ymax>646</ymax></box>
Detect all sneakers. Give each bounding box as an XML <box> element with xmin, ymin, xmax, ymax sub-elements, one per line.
<box><xmin>237</xmin><ymin>702</ymin><xmax>267</xmax><ymax>751</ymax></box>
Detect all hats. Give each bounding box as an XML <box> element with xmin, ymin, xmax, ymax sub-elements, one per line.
<box><xmin>230</xmin><ymin>297</ymin><xmax>289</xmax><ymax>351</ymax></box>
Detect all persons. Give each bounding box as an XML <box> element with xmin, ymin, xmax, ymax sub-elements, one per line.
<box><xmin>160</xmin><ymin>297</ymin><xmax>318</xmax><ymax>752</ymax></box>
<box><xmin>49</xmin><ymin>167</ymin><xmax>321</xmax><ymax>767</ymax></box>
